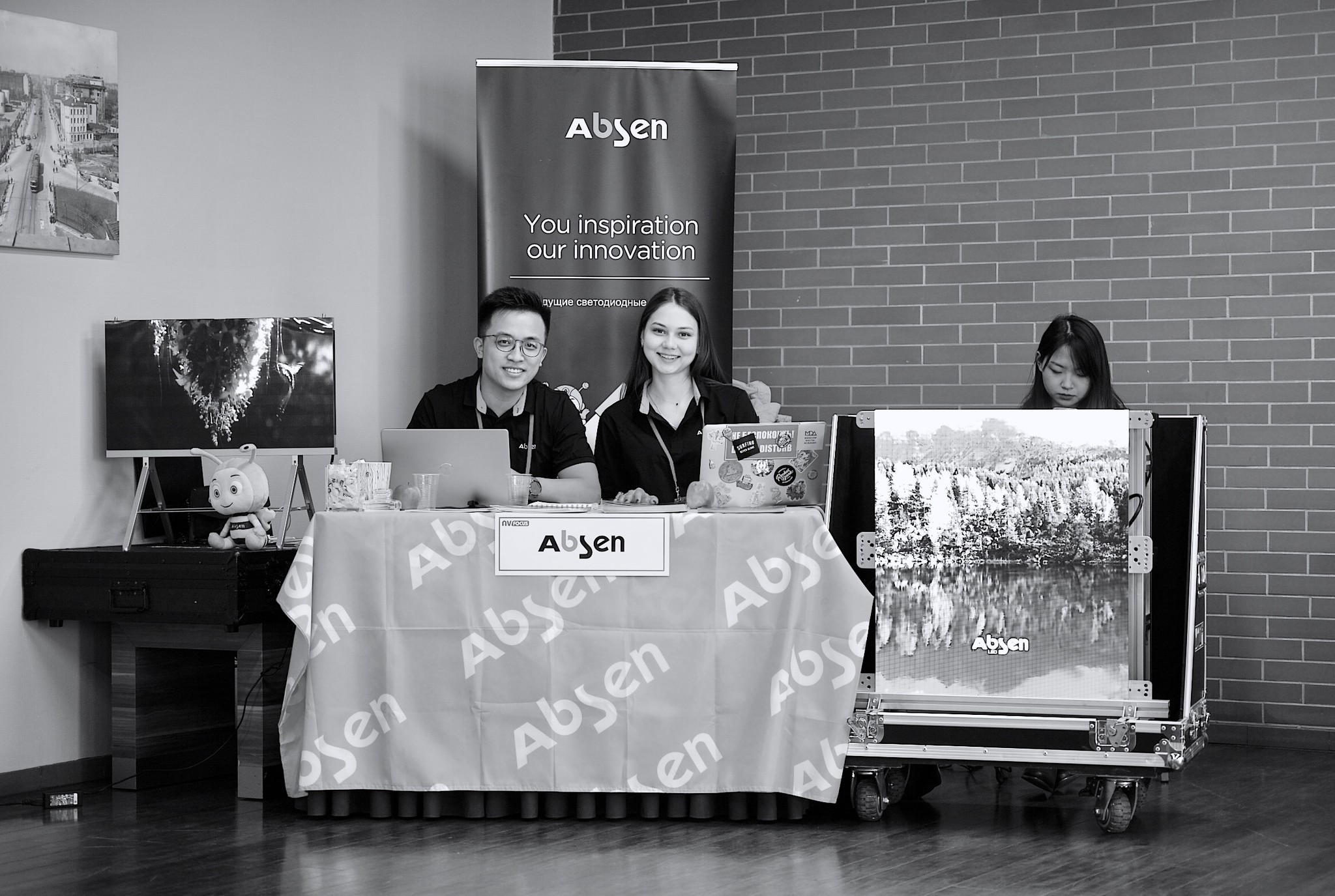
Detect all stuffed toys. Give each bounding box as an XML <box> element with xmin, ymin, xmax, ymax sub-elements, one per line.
<box><xmin>190</xmin><ymin>444</ymin><xmax>276</xmax><ymax>550</ymax></box>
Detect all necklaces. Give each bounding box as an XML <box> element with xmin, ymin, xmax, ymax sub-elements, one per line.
<box><xmin>643</xmin><ymin>385</ymin><xmax>678</xmax><ymax>430</ymax></box>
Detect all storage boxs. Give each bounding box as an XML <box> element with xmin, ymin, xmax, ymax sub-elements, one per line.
<box><xmin>826</xmin><ymin>409</ymin><xmax>1212</xmax><ymax>832</ymax></box>
<box><xmin>21</xmin><ymin>539</ymin><xmax>302</xmax><ymax>624</ymax></box>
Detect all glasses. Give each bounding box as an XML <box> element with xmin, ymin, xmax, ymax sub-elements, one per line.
<box><xmin>482</xmin><ymin>333</ymin><xmax>545</xmax><ymax>357</ymax></box>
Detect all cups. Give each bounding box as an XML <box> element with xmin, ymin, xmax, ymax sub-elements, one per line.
<box><xmin>504</xmin><ymin>473</ymin><xmax>532</xmax><ymax>509</ymax></box>
<box><xmin>412</xmin><ymin>473</ymin><xmax>440</xmax><ymax>511</ymax></box>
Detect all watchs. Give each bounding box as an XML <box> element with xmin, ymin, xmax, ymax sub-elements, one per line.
<box><xmin>528</xmin><ymin>474</ymin><xmax>540</xmax><ymax>503</ymax></box>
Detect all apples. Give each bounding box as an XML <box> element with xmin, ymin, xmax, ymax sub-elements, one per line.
<box><xmin>686</xmin><ymin>481</ymin><xmax>714</xmax><ymax>509</ymax></box>
<box><xmin>393</xmin><ymin>482</ymin><xmax>421</xmax><ymax>509</ymax></box>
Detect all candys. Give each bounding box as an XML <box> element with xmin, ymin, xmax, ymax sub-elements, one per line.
<box><xmin>325</xmin><ymin>458</ymin><xmax>363</xmax><ymax>510</ymax></box>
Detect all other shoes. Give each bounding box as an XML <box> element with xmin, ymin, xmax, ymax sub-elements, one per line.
<box><xmin>1020</xmin><ymin>762</ymin><xmax>1079</xmax><ymax>794</ymax></box>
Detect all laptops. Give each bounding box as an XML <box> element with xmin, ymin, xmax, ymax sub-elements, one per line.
<box><xmin>381</xmin><ymin>429</ymin><xmax>511</xmax><ymax>508</ymax></box>
<box><xmin>698</xmin><ymin>422</ymin><xmax>825</xmax><ymax>509</ymax></box>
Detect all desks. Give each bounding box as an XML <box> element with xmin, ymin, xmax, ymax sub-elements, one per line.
<box><xmin>276</xmin><ymin>506</ymin><xmax>876</xmax><ymax>821</ymax></box>
<box><xmin>110</xmin><ymin>615</ymin><xmax>293</xmax><ymax>800</ymax></box>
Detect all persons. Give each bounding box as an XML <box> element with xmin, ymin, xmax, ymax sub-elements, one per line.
<box><xmin>1020</xmin><ymin>314</ymin><xmax>1125</xmax><ymax>796</ymax></box>
<box><xmin>593</xmin><ymin>288</ymin><xmax>761</xmax><ymax>508</ymax></box>
<box><xmin>408</xmin><ymin>289</ymin><xmax>600</xmax><ymax>509</ymax></box>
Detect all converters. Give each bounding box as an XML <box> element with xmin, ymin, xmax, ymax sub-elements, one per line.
<box><xmin>41</xmin><ymin>790</ymin><xmax>84</xmax><ymax>809</ymax></box>
